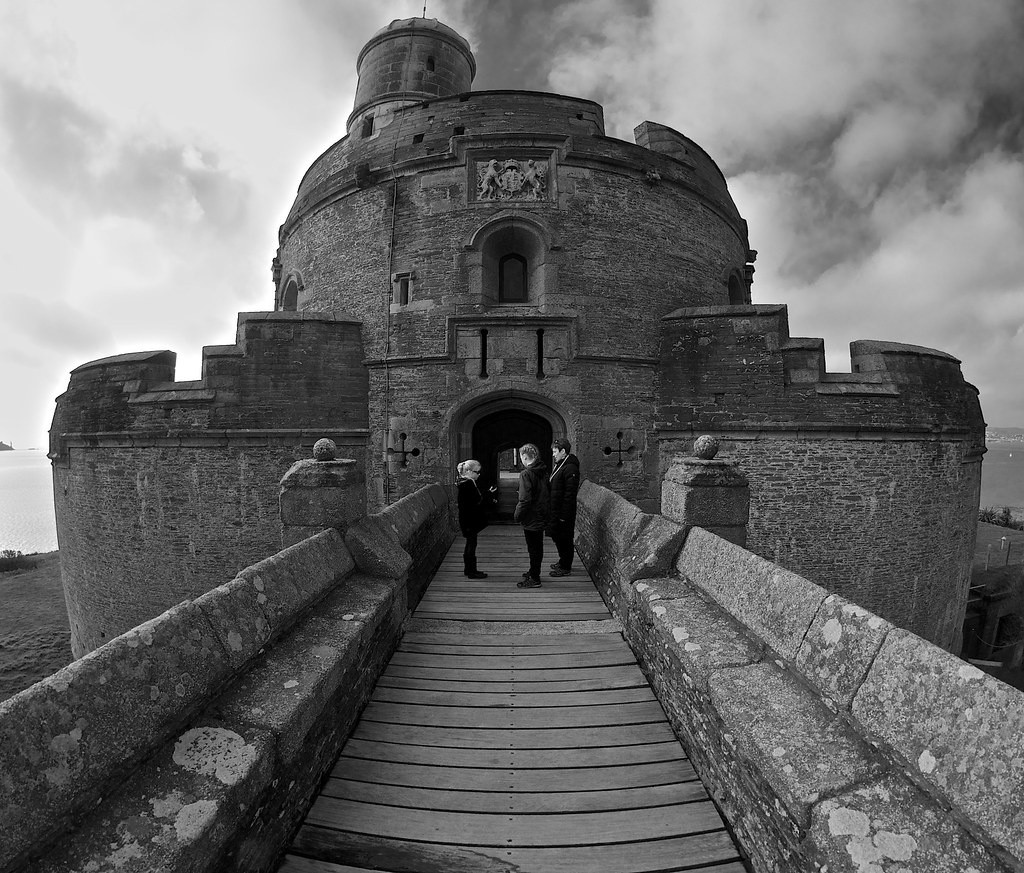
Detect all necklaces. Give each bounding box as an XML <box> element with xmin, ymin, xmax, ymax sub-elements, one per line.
<box><xmin>549</xmin><ymin>455</ymin><xmax>569</xmax><ymax>481</ymax></box>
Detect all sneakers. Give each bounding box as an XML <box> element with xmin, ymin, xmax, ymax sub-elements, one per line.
<box><xmin>549</xmin><ymin>568</ymin><xmax>571</xmax><ymax>577</ymax></box>
<box><xmin>516</xmin><ymin>569</ymin><xmax>542</xmax><ymax>588</ymax></box>
<box><xmin>550</xmin><ymin>562</ymin><xmax>560</xmax><ymax>569</ymax></box>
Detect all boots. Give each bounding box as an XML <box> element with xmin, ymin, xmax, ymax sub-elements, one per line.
<box><xmin>463</xmin><ymin>554</ymin><xmax>483</xmax><ymax>575</ymax></box>
<box><xmin>465</xmin><ymin>555</ymin><xmax>488</xmax><ymax>579</ymax></box>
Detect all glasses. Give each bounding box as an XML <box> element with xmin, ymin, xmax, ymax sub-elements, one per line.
<box><xmin>470</xmin><ymin>470</ymin><xmax>480</xmax><ymax>474</ymax></box>
<box><xmin>554</xmin><ymin>440</ymin><xmax>562</xmax><ymax>446</ymax></box>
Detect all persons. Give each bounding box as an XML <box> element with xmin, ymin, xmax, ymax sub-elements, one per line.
<box><xmin>548</xmin><ymin>437</ymin><xmax>581</xmax><ymax>576</ymax></box>
<box><xmin>521</xmin><ymin>158</ymin><xmax>544</xmax><ymax>197</ymax></box>
<box><xmin>479</xmin><ymin>159</ymin><xmax>503</xmax><ymax>199</ymax></box>
<box><xmin>457</xmin><ymin>459</ymin><xmax>499</xmax><ymax>579</ymax></box>
<box><xmin>513</xmin><ymin>442</ymin><xmax>549</xmax><ymax>589</ymax></box>
